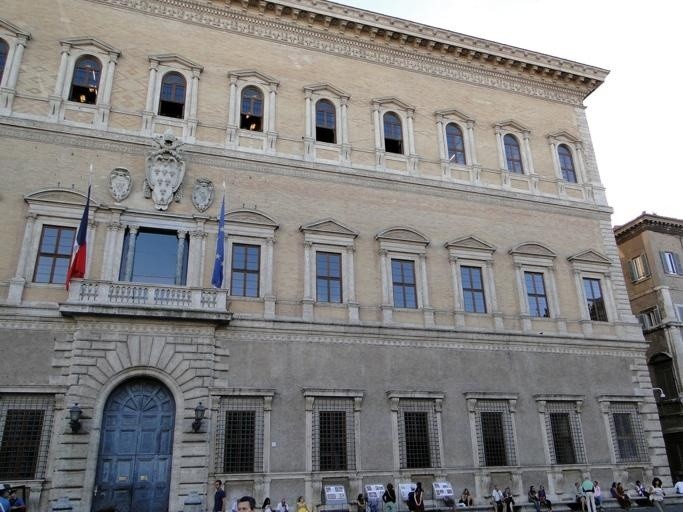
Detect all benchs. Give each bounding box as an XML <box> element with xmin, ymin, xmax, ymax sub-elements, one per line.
<box><xmin>226</xmin><ymin>479</ymin><xmax>683</xmax><ymax>511</ymax></box>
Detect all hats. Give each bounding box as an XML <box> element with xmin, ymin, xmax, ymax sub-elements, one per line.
<box><xmin>0</xmin><ymin>483</ymin><xmax>10</xmax><ymax>490</ymax></box>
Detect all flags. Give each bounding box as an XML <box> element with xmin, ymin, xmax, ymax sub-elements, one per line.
<box><xmin>208</xmin><ymin>194</ymin><xmax>224</xmax><ymax>289</ymax></box>
<box><xmin>64</xmin><ymin>183</ymin><xmax>91</xmax><ymax>291</ymax></box>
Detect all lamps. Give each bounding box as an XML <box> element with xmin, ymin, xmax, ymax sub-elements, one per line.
<box><xmin>64</xmin><ymin>401</ymin><xmax>92</xmax><ymax>431</ymax></box>
<box><xmin>191</xmin><ymin>401</ymin><xmax>206</xmax><ymax>431</ymax></box>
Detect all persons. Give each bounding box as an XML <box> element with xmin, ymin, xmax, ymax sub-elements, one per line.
<box><xmin>381</xmin><ymin>482</ymin><xmax>396</xmax><ymax>512</ymax></box>
<box><xmin>231</xmin><ymin>497</ymin><xmax>241</xmax><ymax>512</ymax></box>
<box><xmin>261</xmin><ymin>496</ymin><xmax>270</xmax><ymax>511</ymax></box>
<box><xmin>356</xmin><ymin>493</ymin><xmax>365</xmax><ymax>512</ymax></box>
<box><xmin>294</xmin><ymin>495</ymin><xmax>310</xmax><ymax>511</ymax></box>
<box><xmin>8</xmin><ymin>488</ymin><xmax>26</xmax><ymax>511</ymax></box>
<box><xmin>212</xmin><ymin>479</ymin><xmax>226</xmax><ymax>511</ymax></box>
<box><xmin>406</xmin><ymin>485</ymin><xmax>415</xmax><ymax>511</ymax></box>
<box><xmin>412</xmin><ymin>480</ymin><xmax>424</xmax><ymax>511</ymax></box>
<box><xmin>237</xmin><ymin>496</ymin><xmax>255</xmax><ymax>511</ymax></box>
<box><xmin>363</xmin><ymin>496</ymin><xmax>371</xmax><ymax>512</ymax></box>
<box><xmin>276</xmin><ymin>497</ymin><xmax>287</xmax><ymax>511</ymax></box>
<box><xmin>458</xmin><ymin>476</ymin><xmax>683</xmax><ymax>511</ymax></box>
<box><xmin>0</xmin><ymin>483</ymin><xmax>12</xmax><ymax>512</ymax></box>
